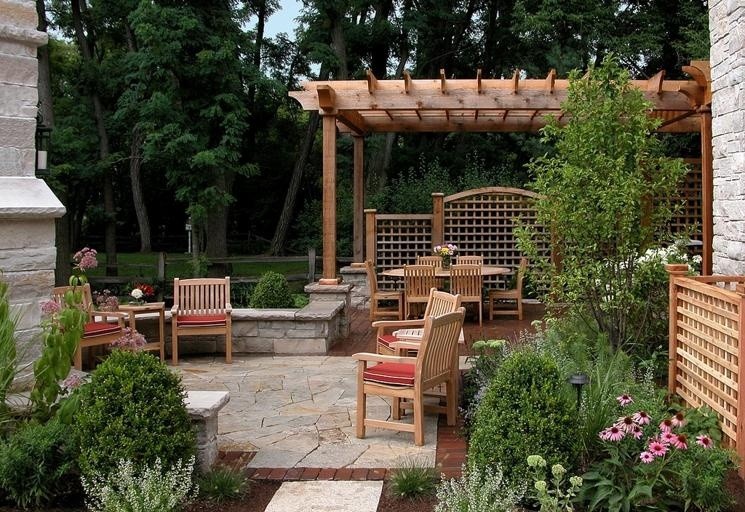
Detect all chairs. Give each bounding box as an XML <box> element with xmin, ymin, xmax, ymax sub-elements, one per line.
<box><xmin>170</xmin><ymin>275</ymin><xmax>235</xmax><ymax>367</ymax></box>
<box><xmin>49</xmin><ymin>281</ymin><xmax>129</xmax><ymax>373</ymax></box>
<box><xmin>351</xmin><ymin>253</ymin><xmax>533</xmax><ymax>446</ymax></box>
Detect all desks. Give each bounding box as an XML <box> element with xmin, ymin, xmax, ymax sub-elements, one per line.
<box><xmin>101</xmin><ymin>301</ymin><xmax>169</xmax><ymax>367</ymax></box>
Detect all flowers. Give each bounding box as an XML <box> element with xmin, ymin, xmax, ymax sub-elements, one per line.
<box><xmin>431</xmin><ymin>242</ymin><xmax>459</xmax><ymax>268</ymax></box>
<box><xmin>125</xmin><ymin>281</ymin><xmax>156</xmax><ymax>300</ymax></box>
